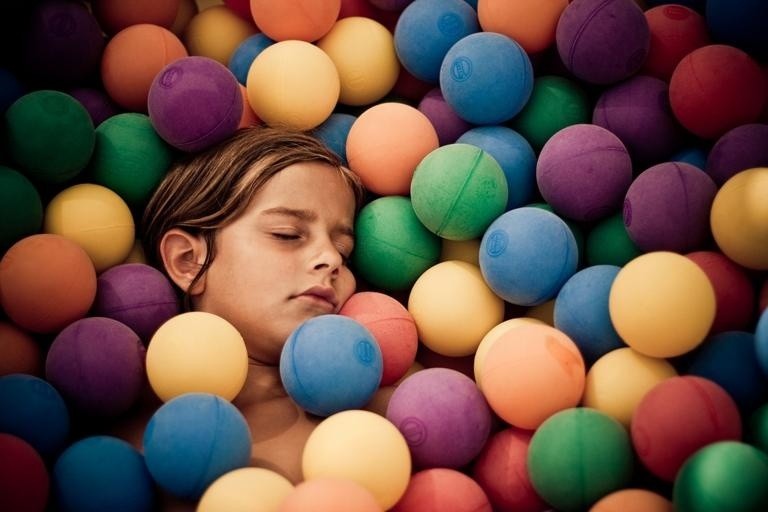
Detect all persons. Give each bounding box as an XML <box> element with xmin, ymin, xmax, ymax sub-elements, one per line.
<box><xmin>118</xmin><ymin>124</ymin><xmax>426</xmax><ymax>512</ymax></box>
